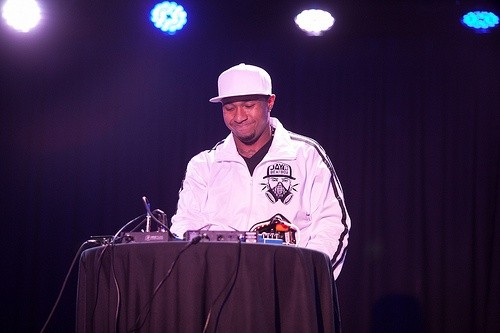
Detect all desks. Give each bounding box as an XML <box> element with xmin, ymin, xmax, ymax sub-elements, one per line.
<box><xmin>76</xmin><ymin>241</ymin><xmax>341</xmax><ymax>333</ymax></box>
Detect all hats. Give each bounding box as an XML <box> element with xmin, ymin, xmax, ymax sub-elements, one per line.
<box><xmin>209</xmin><ymin>62</ymin><xmax>273</xmax><ymax>102</ymax></box>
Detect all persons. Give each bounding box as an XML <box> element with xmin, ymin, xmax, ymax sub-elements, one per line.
<box><xmin>170</xmin><ymin>63</ymin><xmax>352</xmax><ymax>281</ymax></box>
